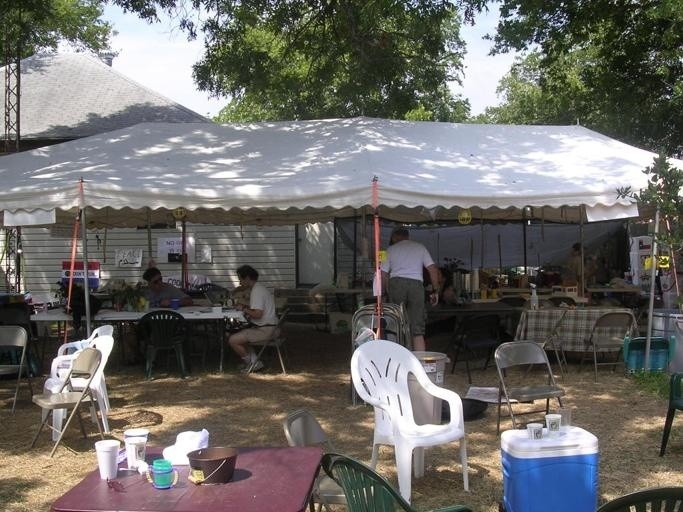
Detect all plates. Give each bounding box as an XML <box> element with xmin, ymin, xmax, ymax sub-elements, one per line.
<box><xmin>201</xmin><ymin>307</ymin><xmax>234</xmax><ymax>314</ymax></box>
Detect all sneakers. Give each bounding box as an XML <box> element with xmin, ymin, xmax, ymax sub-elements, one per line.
<box><xmin>237</xmin><ymin>360</ymin><xmax>252</xmax><ymax>369</ymax></box>
<box><xmin>242</xmin><ymin>362</ymin><xmax>264</xmax><ymax>373</ymax></box>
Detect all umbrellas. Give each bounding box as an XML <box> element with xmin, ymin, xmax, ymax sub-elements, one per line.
<box><xmin>0</xmin><ymin>115</ymin><xmax>683</xmax><ymax>377</ymax></box>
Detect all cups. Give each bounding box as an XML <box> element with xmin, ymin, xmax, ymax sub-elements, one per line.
<box><xmin>172</xmin><ymin>298</ymin><xmax>181</xmax><ymax>311</ymax></box>
<box><xmin>212</xmin><ymin>307</ymin><xmax>224</xmax><ymax>318</ymax></box>
<box><xmin>526</xmin><ymin>423</ymin><xmax>543</xmax><ymax>443</ymax></box>
<box><xmin>123</xmin><ymin>428</ymin><xmax>149</xmax><ymax>470</ymax></box>
<box><xmin>95</xmin><ymin>440</ymin><xmax>119</xmax><ymax>480</ymax></box>
<box><xmin>545</xmin><ymin>414</ymin><xmax>561</xmax><ymax>437</ymax></box>
<box><xmin>558</xmin><ymin>407</ymin><xmax>571</xmax><ymax>431</ymax></box>
<box><xmin>145</xmin><ymin>459</ymin><xmax>181</xmax><ymax>490</ymax></box>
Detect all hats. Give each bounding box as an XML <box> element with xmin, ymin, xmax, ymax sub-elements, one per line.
<box><xmin>389</xmin><ymin>227</ymin><xmax>408</xmax><ymax>245</ymax></box>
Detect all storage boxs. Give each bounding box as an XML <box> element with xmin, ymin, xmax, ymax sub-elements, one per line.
<box><xmin>499</xmin><ymin>424</ymin><xmax>600</xmax><ymax>512</ymax></box>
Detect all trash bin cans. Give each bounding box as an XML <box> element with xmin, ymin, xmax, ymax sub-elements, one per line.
<box><xmin>403</xmin><ymin>350</ymin><xmax>452</xmax><ymax>425</ymax></box>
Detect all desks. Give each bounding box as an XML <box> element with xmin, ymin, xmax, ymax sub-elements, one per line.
<box><xmin>30</xmin><ymin>304</ymin><xmax>244</xmax><ymax>375</ymax></box>
<box><xmin>585</xmin><ymin>288</ymin><xmax>642</xmax><ymax>308</ymax></box>
<box><xmin>515</xmin><ymin>303</ymin><xmax>640</xmax><ymax>368</ymax></box>
<box><xmin>503</xmin><ymin>294</ymin><xmax>588</xmax><ymax>309</ymax></box>
<box><xmin>416</xmin><ymin>303</ymin><xmax>527</xmax><ymax>348</ymax></box>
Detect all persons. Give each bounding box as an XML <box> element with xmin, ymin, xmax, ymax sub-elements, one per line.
<box><xmin>381</xmin><ymin>228</ymin><xmax>439</xmax><ymax>351</ymax></box>
<box><xmin>139</xmin><ymin>266</ymin><xmax>192</xmax><ymax>307</ymax></box>
<box><xmin>228</xmin><ymin>265</ymin><xmax>280</xmax><ymax>372</ymax></box>
<box><xmin>425</xmin><ymin>267</ymin><xmax>456</xmax><ymax>305</ymax></box>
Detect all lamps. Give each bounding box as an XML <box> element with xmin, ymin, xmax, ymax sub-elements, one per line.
<box><xmin>457</xmin><ymin>209</ymin><xmax>473</xmax><ymax>226</ymax></box>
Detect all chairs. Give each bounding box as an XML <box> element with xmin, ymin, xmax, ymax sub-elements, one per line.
<box><xmin>351</xmin><ymin>302</ymin><xmax>413</xmax><ymax>407</ymax></box>
<box><xmin>0</xmin><ymin>323</ymin><xmax>34</xmax><ymax>412</ymax></box>
<box><xmin>598</xmin><ymin>486</ymin><xmax>683</xmax><ymax>512</ymax></box>
<box><xmin>321</xmin><ymin>452</ymin><xmax>474</xmax><ymax>512</ymax></box>
<box><xmin>242</xmin><ymin>308</ymin><xmax>291</xmax><ymax>377</ymax></box>
<box><xmin>56</xmin><ymin>324</ymin><xmax>113</xmax><ymax>415</ymax></box>
<box><xmin>494</xmin><ymin>341</ymin><xmax>566</xmax><ymax>436</ymax></box>
<box><xmin>31</xmin><ymin>347</ymin><xmax>109</xmax><ymax>456</ymax></box>
<box><xmin>349</xmin><ymin>339</ymin><xmax>470</xmax><ymax>504</ymax></box>
<box><xmin>281</xmin><ymin>409</ymin><xmax>390</xmax><ymax>512</ymax></box>
<box><xmin>135</xmin><ymin>310</ymin><xmax>190</xmax><ymax>381</ymax></box>
<box><xmin>656</xmin><ymin>369</ymin><xmax>683</xmax><ymax>456</ymax></box>
<box><xmin>42</xmin><ymin>335</ymin><xmax>114</xmax><ymax>437</ymax></box>
<box><xmin>523</xmin><ymin>309</ymin><xmax>569</xmax><ymax>385</ymax></box>
<box><xmin>580</xmin><ymin>311</ymin><xmax>634</xmax><ymax>383</ymax></box>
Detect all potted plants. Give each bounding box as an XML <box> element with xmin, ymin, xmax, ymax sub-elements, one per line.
<box><xmin>614</xmin><ymin>154</ymin><xmax>682</xmax><ymax>374</ymax></box>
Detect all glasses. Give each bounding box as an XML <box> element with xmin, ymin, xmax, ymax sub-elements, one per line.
<box><xmin>149</xmin><ymin>277</ymin><xmax>163</xmax><ymax>283</ymax></box>
